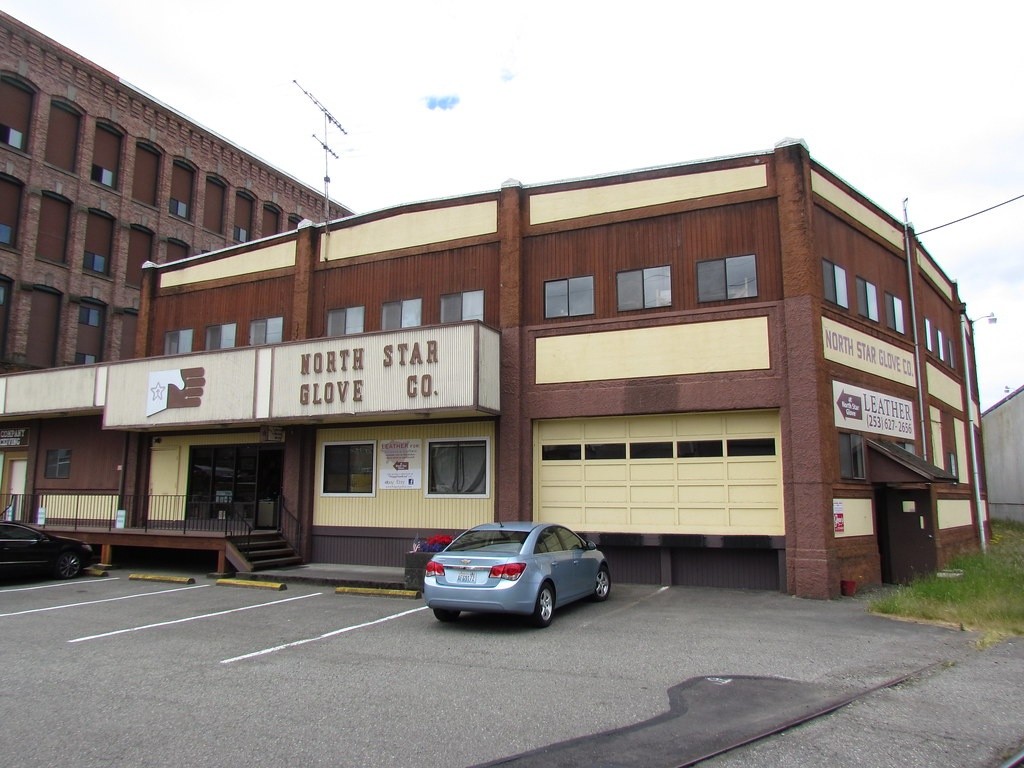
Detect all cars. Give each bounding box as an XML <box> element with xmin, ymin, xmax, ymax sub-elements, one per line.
<box><xmin>0</xmin><ymin>520</ymin><xmax>95</xmax><ymax>581</ymax></box>
<box><xmin>423</xmin><ymin>521</ymin><xmax>612</xmax><ymax>628</ymax></box>
